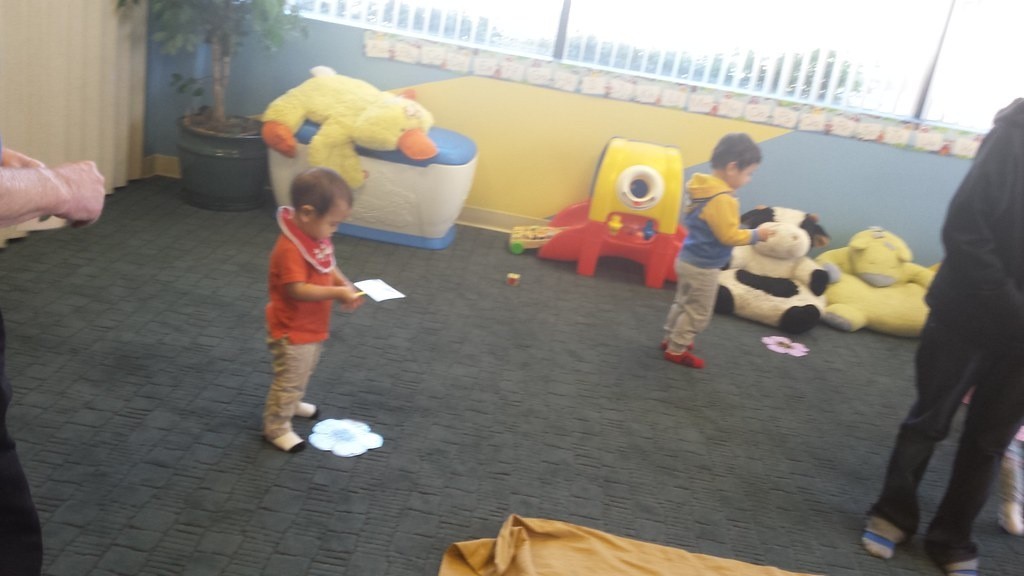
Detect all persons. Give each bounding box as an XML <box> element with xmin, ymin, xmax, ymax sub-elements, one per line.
<box><xmin>261</xmin><ymin>167</ymin><xmax>366</xmax><ymax>455</ymax></box>
<box><xmin>999</xmin><ymin>424</ymin><xmax>1023</xmax><ymax>538</ymax></box>
<box><xmin>859</xmin><ymin>97</ymin><xmax>1023</xmax><ymax>576</ymax></box>
<box><xmin>0</xmin><ymin>147</ymin><xmax>105</xmax><ymax>575</ymax></box>
<box><xmin>659</xmin><ymin>132</ymin><xmax>777</xmax><ymax>370</ymax></box>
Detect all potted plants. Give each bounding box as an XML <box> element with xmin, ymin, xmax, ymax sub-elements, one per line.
<box><xmin>115</xmin><ymin>0</ymin><xmax>309</xmax><ymax>212</ymax></box>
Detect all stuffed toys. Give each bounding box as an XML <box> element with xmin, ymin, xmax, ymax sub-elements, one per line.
<box><xmin>715</xmin><ymin>203</ymin><xmax>830</xmax><ymax>335</ymax></box>
<box><xmin>259</xmin><ymin>63</ymin><xmax>441</xmax><ymax>189</ymax></box>
<box><xmin>814</xmin><ymin>226</ymin><xmax>939</xmax><ymax>341</ymax></box>
